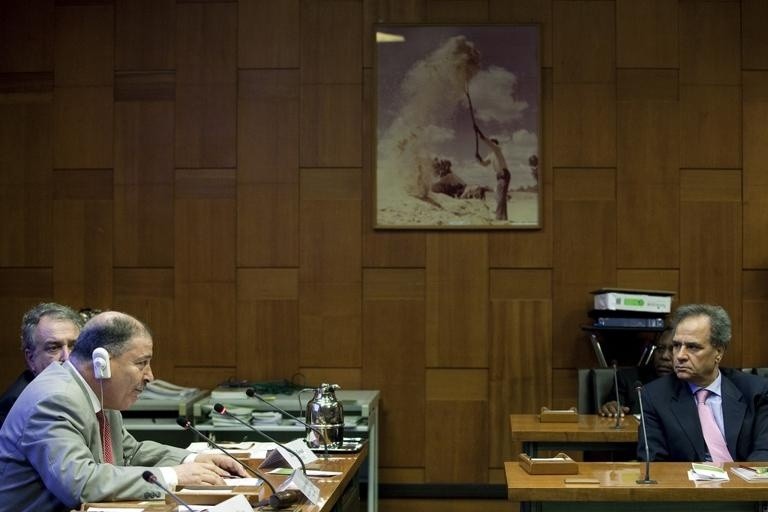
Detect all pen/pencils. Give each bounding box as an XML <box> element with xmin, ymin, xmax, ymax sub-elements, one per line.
<box><xmin>220</xmin><ymin>475</ymin><xmax>236</xmax><ymax>478</ymax></box>
<box><xmin>739</xmin><ymin>465</ymin><xmax>757</xmax><ymax>471</ymax></box>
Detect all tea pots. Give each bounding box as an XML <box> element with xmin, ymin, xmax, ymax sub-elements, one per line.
<box><xmin>298</xmin><ymin>384</ymin><xmax>343</xmax><ymax>450</ymax></box>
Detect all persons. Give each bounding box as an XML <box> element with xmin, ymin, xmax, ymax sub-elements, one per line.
<box><xmin>636</xmin><ymin>304</ymin><xmax>768</xmax><ymax>462</ymax></box>
<box><xmin>583</xmin><ymin>327</ymin><xmax>674</xmax><ymax>461</ymax></box>
<box><xmin>0</xmin><ymin>302</ymin><xmax>84</xmax><ymax>429</ymax></box>
<box><xmin>0</xmin><ymin>310</ymin><xmax>251</xmax><ymax>512</ymax></box>
<box><xmin>476</xmin><ymin>127</ymin><xmax>510</xmax><ymax>220</ymax></box>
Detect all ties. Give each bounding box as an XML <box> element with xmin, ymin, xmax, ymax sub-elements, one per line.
<box><xmin>96</xmin><ymin>411</ymin><xmax>114</xmax><ymax>464</ymax></box>
<box><xmin>696</xmin><ymin>389</ymin><xmax>734</xmax><ymax>462</ymax></box>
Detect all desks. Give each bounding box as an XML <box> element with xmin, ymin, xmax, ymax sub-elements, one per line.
<box><xmin>120</xmin><ymin>386</ymin><xmax>381</xmax><ymax>512</ymax></box>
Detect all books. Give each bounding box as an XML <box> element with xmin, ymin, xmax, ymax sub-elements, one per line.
<box><xmin>211</xmin><ymin>386</ymin><xmax>274</xmax><ymax>427</ymax></box>
<box><xmin>730</xmin><ymin>466</ymin><xmax>768</xmax><ymax>483</ymax></box>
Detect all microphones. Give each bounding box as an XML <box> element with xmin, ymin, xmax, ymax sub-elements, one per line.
<box><xmin>142</xmin><ymin>471</ymin><xmax>194</xmax><ymax>512</ymax></box>
<box><xmin>634</xmin><ymin>380</ymin><xmax>656</xmax><ymax>484</ymax></box>
<box><xmin>175</xmin><ymin>416</ymin><xmax>275</xmax><ymax>495</ymax></box>
<box><xmin>213</xmin><ymin>402</ymin><xmax>306</xmax><ymax>474</ymax></box>
<box><xmin>244</xmin><ymin>388</ymin><xmax>328</xmax><ymax>460</ymax></box>
<box><xmin>609</xmin><ymin>359</ymin><xmax>623</xmax><ymax>429</ymax></box>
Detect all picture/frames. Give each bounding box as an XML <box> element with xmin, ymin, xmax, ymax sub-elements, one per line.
<box><xmin>371</xmin><ymin>22</ymin><xmax>546</xmax><ymax>232</ymax></box>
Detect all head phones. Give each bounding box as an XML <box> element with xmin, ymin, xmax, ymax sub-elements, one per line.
<box><xmin>92</xmin><ymin>347</ymin><xmax>112</xmax><ymax>380</ymax></box>
